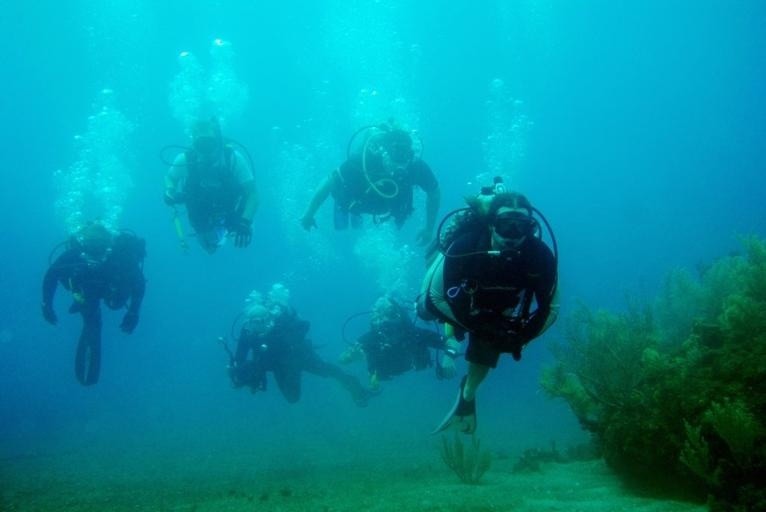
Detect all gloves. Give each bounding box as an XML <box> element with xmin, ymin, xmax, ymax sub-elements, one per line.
<box><xmin>119</xmin><ymin>312</ymin><xmax>139</xmax><ymax>333</ymax></box>
<box><xmin>232</xmin><ymin>217</ymin><xmax>252</xmax><ymax>247</ymax></box>
<box><xmin>165</xmin><ymin>191</ymin><xmax>175</xmax><ymax>206</ymax></box>
<box><xmin>41</xmin><ymin>302</ymin><xmax>58</xmax><ymax>326</ymax></box>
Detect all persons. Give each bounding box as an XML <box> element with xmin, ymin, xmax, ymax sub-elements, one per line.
<box><xmin>162</xmin><ymin>115</ymin><xmax>258</xmax><ymax>254</ymax></box>
<box><xmin>336</xmin><ymin>294</ymin><xmax>460</xmax><ymax>397</ymax></box>
<box><xmin>41</xmin><ymin>220</ymin><xmax>146</xmax><ymax>387</ymax></box>
<box><xmin>215</xmin><ymin>303</ymin><xmax>368</xmax><ymax>409</ymax></box>
<box><xmin>300</xmin><ymin>130</ymin><xmax>441</xmax><ymax>248</ymax></box>
<box><xmin>414</xmin><ymin>190</ymin><xmax>559</xmax><ymax>436</ymax></box>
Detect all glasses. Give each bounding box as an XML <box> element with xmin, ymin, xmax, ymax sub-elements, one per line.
<box><xmin>388</xmin><ymin>146</ymin><xmax>413</xmax><ymax>162</ymax></box>
<box><xmin>85</xmin><ymin>242</ymin><xmax>106</xmax><ymax>255</ymax></box>
<box><xmin>494</xmin><ymin>212</ymin><xmax>533</xmax><ymax>238</ymax></box>
<box><xmin>194</xmin><ymin>137</ymin><xmax>217</xmax><ymax>151</ymax></box>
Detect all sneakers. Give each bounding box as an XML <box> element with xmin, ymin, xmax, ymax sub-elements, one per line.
<box><xmin>342</xmin><ymin>376</ymin><xmax>360</xmax><ymax>394</ymax></box>
<box><xmin>82</xmin><ymin>319</ymin><xmax>102</xmax><ymax>344</ymax></box>
<box><xmin>456</xmin><ymin>376</ymin><xmax>475</xmax><ymax>415</ymax></box>
